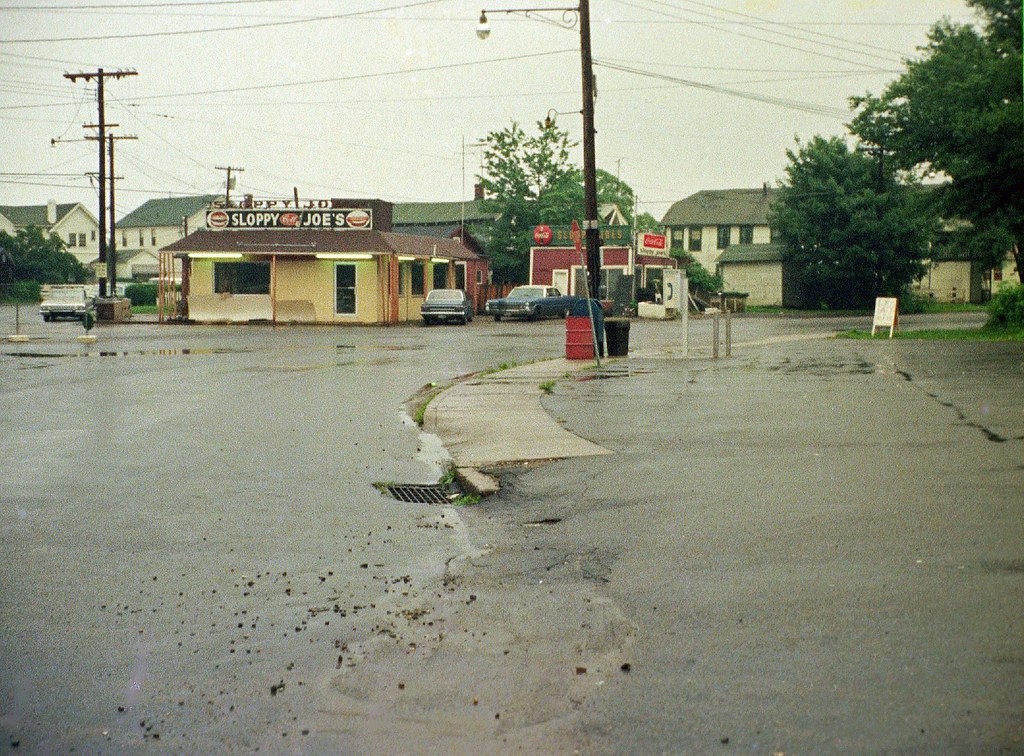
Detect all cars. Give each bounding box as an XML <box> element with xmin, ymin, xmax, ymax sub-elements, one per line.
<box><xmin>421</xmin><ymin>288</ymin><xmax>473</xmax><ymax>323</ymax></box>
<box><xmin>37</xmin><ymin>289</ymin><xmax>94</xmax><ymax>321</ymax></box>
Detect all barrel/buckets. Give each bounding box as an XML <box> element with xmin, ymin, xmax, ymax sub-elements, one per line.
<box><xmin>605</xmin><ymin>321</ymin><xmax>630</xmax><ymax>355</ymax></box>
<box><xmin>566</xmin><ymin>316</ymin><xmax>593</xmax><ymax>360</ymax></box>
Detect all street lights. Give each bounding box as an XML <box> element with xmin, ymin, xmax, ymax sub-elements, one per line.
<box><xmin>476</xmin><ymin>0</ymin><xmax>606</xmax><ymax>301</ymax></box>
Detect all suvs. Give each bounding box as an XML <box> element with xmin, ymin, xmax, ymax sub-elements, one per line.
<box><xmin>485</xmin><ymin>285</ymin><xmax>573</xmax><ymax>322</ymax></box>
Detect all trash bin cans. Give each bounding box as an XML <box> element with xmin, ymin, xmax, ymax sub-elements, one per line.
<box><xmin>603</xmin><ymin>319</ymin><xmax>631</xmax><ymax>356</ymax></box>
<box><xmin>572</xmin><ymin>299</ymin><xmax>604</xmax><ymax>357</ymax></box>
<box><xmin>566</xmin><ymin>316</ymin><xmax>594</xmax><ymax>360</ymax></box>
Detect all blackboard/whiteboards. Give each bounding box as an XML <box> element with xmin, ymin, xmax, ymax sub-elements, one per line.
<box><xmin>873</xmin><ymin>296</ymin><xmax>898</xmax><ymax>327</ymax></box>
